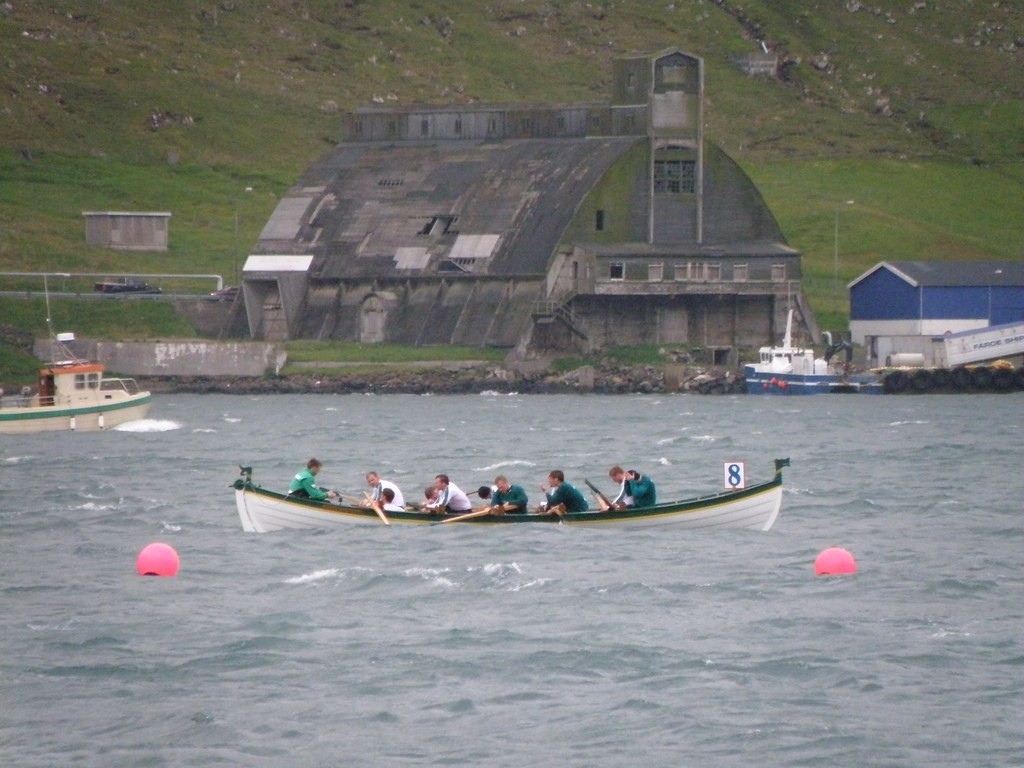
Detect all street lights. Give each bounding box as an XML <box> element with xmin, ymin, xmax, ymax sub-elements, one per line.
<box><xmin>234</xmin><ymin>185</ymin><xmax>253</xmax><ymax>289</ymax></box>
<box><xmin>833</xmin><ymin>200</ymin><xmax>855</xmax><ymax>311</ymax></box>
<box><xmin>987</xmin><ymin>269</ymin><xmax>1003</xmax><ymax>325</ymax></box>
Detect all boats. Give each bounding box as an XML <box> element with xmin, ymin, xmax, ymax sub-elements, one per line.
<box><xmin>220</xmin><ymin>458</ymin><xmax>793</xmax><ymax>534</ymax></box>
<box><xmin>1</xmin><ymin>274</ymin><xmax>154</xmax><ymax>435</ymax></box>
<box><xmin>742</xmin><ymin>310</ymin><xmax>894</xmax><ymax>396</ymax></box>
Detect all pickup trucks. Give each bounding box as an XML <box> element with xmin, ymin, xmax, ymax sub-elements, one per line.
<box><xmin>91</xmin><ymin>276</ymin><xmax>151</xmax><ymax>293</ymax></box>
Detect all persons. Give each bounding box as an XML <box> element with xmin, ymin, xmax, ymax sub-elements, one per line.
<box><xmin>287</xmin><ymin>459</ymin><xmax>336</xmax><ymax>504</ymax></box>
<box><xmin>422</xmin><ymin>474</ymin><xmax>473</xmax><ymax>514</ymax></box>
<box><xmin>477</xmin><ymin>475</ymin><xmax>528</xmax><ymax>514</ymax></box>
<box><xmin>607</xmin><ymin>466</ymin><xmax>656</xmax><ymax>510</ymax></box>
<box><xmin>360</xmin><ymin>471</ymin><xmax>406</xmax><ymax>513</ymax></box>
<box><xmin>540</xmin><ymin>469</ymin><xmax>590</xmax><ymax>513</ymax></box>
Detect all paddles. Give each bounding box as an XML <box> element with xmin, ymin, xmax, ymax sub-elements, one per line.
<box><xmin>363</xmin><ymin>491</ymin><xmax>390</xmax><ymax>527</ymax></box>
<box><xmin>584</xmin><ymin>478</ymin><xmax>609</xmax><ymax>507</ymax></box>
<box><xmin>430</xmin><ymin>505</ymin><xmax>493</xmax><ymax>527</ymax></box>
